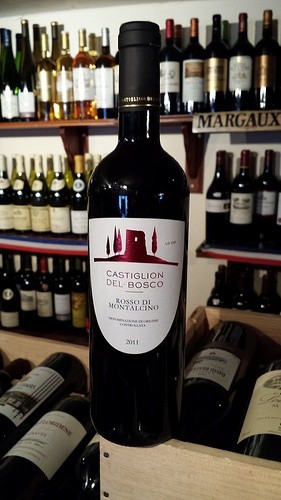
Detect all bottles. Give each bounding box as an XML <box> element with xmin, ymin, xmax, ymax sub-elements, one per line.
<box><xmin>89</xmin><ymin>23</ymin><xmax>190</xmax><ymax>448</ymax></box>
<box><xmin>0</xmin><ymin>19</ymin><xmax>119</xmax><ymax>123</ymax></box>
<box><xmin>205</xmin><ymin>149</ymin><xmax>281</xmax><ymax>255</ymax></box>
<box><xmin>0</xmin><ymin>348</ymin><xmax>100</xmax><ymax>500</ymax></box>
<box><xmin>0</xmin><ymin>251</ymin><xmax>90</xmax><ymax>335</ymax></box>
<box><xmin>159</xmin><ymin>10</ymin><xmax>281</xmax><ymax>116</ymax></box>
<box><xmin>176</xmin><ymin>319</ymin><xmax>280</xmax><ymax>463</ymax></box>
<box><xmin>0</xmin><ymin>153</ymin><xmax>102</xmax><ymax>238</ymax></box>
<box><xmin>206</xmin><ymin>261</ymin><xmax>281</xmax><ymax>315</ymax></box>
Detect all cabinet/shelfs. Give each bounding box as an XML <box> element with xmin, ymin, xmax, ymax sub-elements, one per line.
<box><xmin>0</xmin><ymin>110</ymin><xmax>281</xmax><ymax>406</ymax></box>
